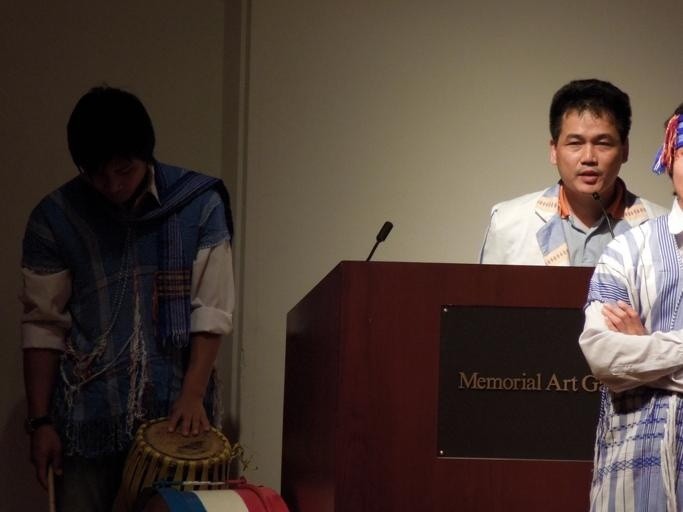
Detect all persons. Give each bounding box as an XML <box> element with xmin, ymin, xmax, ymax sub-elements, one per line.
<box><xmin>579</xmin><ymin>101</ymin><xmax>683</xmax><ymax>512</ymax></box>
<box><xmin>477</xmin><ymin>78</ymin><xmax>672</xmax><ymax>267</ymax></box>
<box><xmin>20</xmin><ymin>86</ymin><xmax>235</xmax><ymax>512</ymax></box>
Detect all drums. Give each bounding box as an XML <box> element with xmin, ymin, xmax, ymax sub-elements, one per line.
<box><xmin>128</xmin><ymin>481</ymin><xmax>289</xmax><ymax>511</ymax></box>
<box><xmin>116</xmin><ymin>417</ymin><xmax>232</xmax><ymax>512</ymax></box>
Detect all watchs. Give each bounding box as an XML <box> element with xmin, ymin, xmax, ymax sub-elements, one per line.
<box><xmin>23</xmin><ymin>414</ymin><xmax>54</xmax><ymax>434</ymax></box>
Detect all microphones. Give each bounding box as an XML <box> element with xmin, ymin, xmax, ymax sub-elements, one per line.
<box><xmin>363</xmin><ymin>221</ymin><xmax>394</xmax><ymax>261</ymax></box>
<box><xmin>591</xmin><ymin>191</ymin><xmax>615</xmax><ymax>237</ymax></box>
